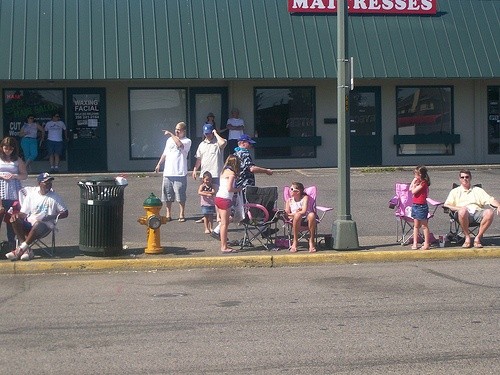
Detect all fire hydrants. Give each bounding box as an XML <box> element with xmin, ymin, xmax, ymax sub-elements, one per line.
<box><xmin>136</xmin><ymin>192</ymin><xmax>169</xmax><ymax>254</ymax></box>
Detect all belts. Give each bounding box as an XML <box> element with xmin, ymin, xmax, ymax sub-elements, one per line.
<box><xmin>25</xmin><ymin>137</ymin><xmax>38</xmax><ymax>139</ymax></box>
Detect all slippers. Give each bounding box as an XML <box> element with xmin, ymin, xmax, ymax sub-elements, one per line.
<box><xmin>224</xmin><ymin>249</ymin><xmax>238</xmax><ymax>253</ymax></box>
<box><xmin>178</xmin><ymin>218</ymin><xmax>185</xmax><ymax>222</ymax></box>
<box><xmin>167</xmin><ymin>217</ymin><xmax>171</xmax><ymax>221</ymax></box>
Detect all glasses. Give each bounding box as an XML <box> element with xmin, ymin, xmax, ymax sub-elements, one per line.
<box><xmin>290</xmin><ymin>187</ymin><xmax>300</xmax><ymax>192</ymax></box>
<box><xmin>460</xmin><ymin>176</ymin><xmax>470</xmax><ymax>179</ymax></box>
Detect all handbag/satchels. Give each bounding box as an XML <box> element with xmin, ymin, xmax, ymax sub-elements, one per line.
<box><xmin>0</xmin><ymin>240</ymin><xmax>16</xmax><ymax>259</ymax></box>
<box><xmin>275</xmin><ymin>239</ymin><xmax>292</xmax><ymax>249</ymax></box>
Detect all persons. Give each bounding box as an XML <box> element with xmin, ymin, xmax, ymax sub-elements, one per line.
<box><xmin>285</xmin><ymin>182</ymin><xmax>317</xmax><ymax>253</ymax></box>
<box><xmin>409</xmin><ymin>166</ymin><xmax>430</xmax><ymax>250</ymax></box>
<box><xmin>45</xmin><ymin>113</ymin><xmax>69</xmax><ymax>173</ymax></box>
<box><xmin>192</xmin><ymin>124</ymin><xmax>228</xmax><ymax>222</ymax></box>
<box><xmin>445</xmin><ymin>171</ymin><xmax>500</xmax><ymax>248</ymax></box>
<box><xmin>210</xmin><ymin>134</ymin><xmax>279</xmax><ymax>241</ymax></box>
<box><xmin>20</xmin><ymin>114</ymin><xmax>44</xmax><ymax>172</ymax></box>
<box><xmin>155</xmin><ymin>122</ymin><xmax>192</xmax><ymax>222</ymax></box>
<box><xmin>0</xmin><ymin>137</ymin><xmax>27</xmax><ymax>251</ymax></box>
<box><xmin>226</xmin><ymin>109</ymin><xmax>245</xmax><ymax>155</ymax></box>
<box><xmin>6</xmin><ymin>172</ymin><xmax>68</xmax><ymax>260</ymax></box>
<box><xmin>198</xmin><ymin>171</ymin><xmax>216</xmax><ymax>233</ymax></box>
<box><xmin>203</xmin><ymin>113</ymin><xmax>215</xmax><ymax>137</ymax></box>
<box><xmin>214</xmin><ymin>155</ymin><xmax>239</xmax><ymax>254</ymax></box>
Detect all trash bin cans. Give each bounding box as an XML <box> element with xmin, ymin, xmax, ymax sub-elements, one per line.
<box><xmin>76</xmin><ymin>177</ymin><xmax>128</xmax><ymax>257</ymax></box>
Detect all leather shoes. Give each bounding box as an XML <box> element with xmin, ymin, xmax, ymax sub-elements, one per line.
<box><xmin>261</xmin><ymin>228</ymin><xmax>279</xmax><ymax>239</ymax></box>
<box><xmin>210</xmin><ymin>231</ymin><xmax>229</xmax><ymax>241</ymax></box>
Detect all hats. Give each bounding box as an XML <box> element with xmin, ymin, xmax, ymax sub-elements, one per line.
<box><xmin>37</xmin><ymin>173</ymin><xmax>54</xmax><ymax>181</ymax></box>
<box><xmin>238</xmin><ymin>134</ymin><xmax>256</xmax><ymax>143</ymax></box>
<box><xmin>203</xmin><ymin>124</ymin><xmax>213</xmax><ymax>133</ymax></box>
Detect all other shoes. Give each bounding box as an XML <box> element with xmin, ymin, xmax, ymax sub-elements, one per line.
<box><xmin>197</xmin><ymin>218</ymin><xmax>204</xmax><ymax>222</ymax></box>
<box><xmin>421</xmin><ymin>244</ymin><xmax>430</xmax><ymax>250</ymax></box>
<box><xmin>6</xmin><ymin>247</ymin><xmax>24</xmax><ymax>260</ymax></box>
<box><xmin>210</xmin><ymin>229</ymin><xmax>213</xmax><ymax>232</ymax></box>
<box><xmin>462</xmin><ymin>242</ymin><xmax>471</xmax><ymax>248</ymax></box>
<box><xmin>205</xmin><ymin>230</ymin><xmax>210</xmax><ymax>233</ymax></box>
<box><xmin>412</xmin><ymin>243</ymin><xmax>418</xmax><ymax>250</ymax></box>
<box><xmin>50</xmin><ymin>168</ymin><xmax>54</xmax><ymax>173</ymax></box>
<box><xmin>21</xmin><ymin>249</ymin><xmax>34</xmax><ymax>261</ymax></box>
<box><xmin>474</xmin><ymin>241</ymin><xmax>482</xmax><ymax>248</ymax></box>
<box><xmin>290</xmin><ymin>246</ymin><xmax>297</xmax><ymax>253</ymax></box>
<box><xmin>54</xmin><ymin>168</ymin><xmax>58</xmax><ymax>172</ymax></box>
<box><xmin>309</xmin><ymin>247</ymin><xmax>316</xmax><ymax>253</ymax></box>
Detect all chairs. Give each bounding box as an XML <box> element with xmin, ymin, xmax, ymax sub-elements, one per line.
<box><xmin>7</xmin><ymin>187</ymin><xmax>58</xmax><ymax>258</ymax></box>
<box><xmin>443</xmin><ymin>183</ymin><xmax>485</xmax><ymax>247</ymax></box>
<box><xmin>278</xmin><ymin>185</ymin><xmax>334</xmax><ymax>251</ymax></box>
<box><xmin>242</xmin><ymin>185</ymin><xmax>280</xmax><ymax>250</ymax></box>
<box><xmin>389</xmin><ymin>183</ymin><xmax>445</xmax><ymax>246</ymax></box>
